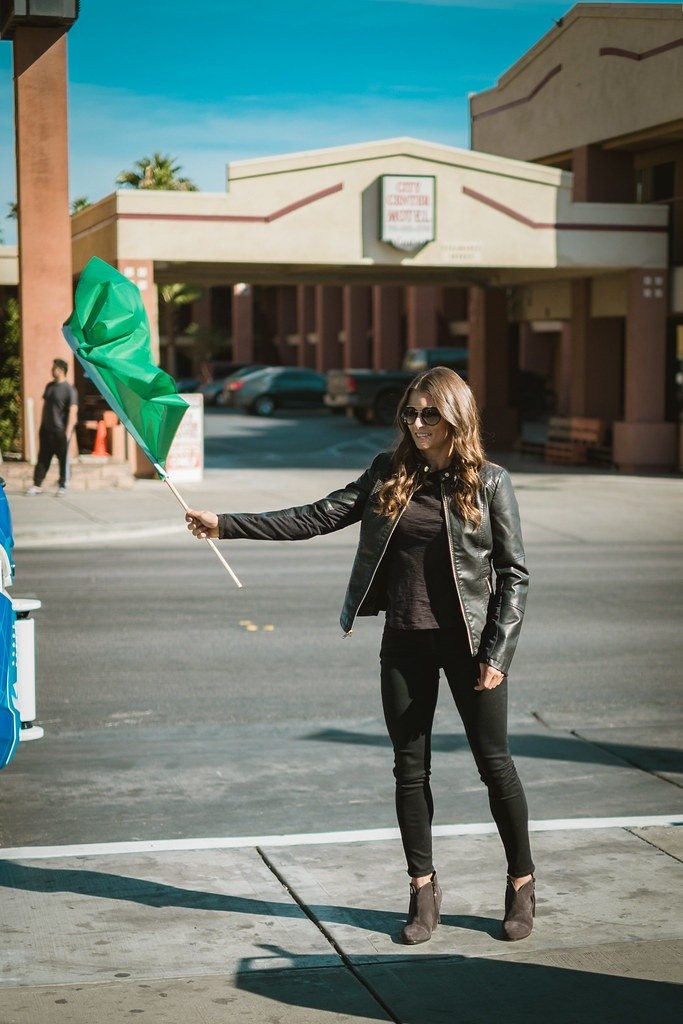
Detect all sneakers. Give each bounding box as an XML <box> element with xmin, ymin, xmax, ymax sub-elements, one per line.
<box><xmin>56</xmin><ymin>487</ymin><xmax>67</xmax><ymax>497</ymax></box>
<box><xmin>25</xmin><ymin>486</ymin><xmax>44</xmax><ymax>495</ymax></box>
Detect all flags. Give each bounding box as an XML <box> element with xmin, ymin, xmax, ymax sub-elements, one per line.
<box><xmin>62</xmin><ymin>256</ymin><xmax>189</xmax><ymax>481</ymax></box>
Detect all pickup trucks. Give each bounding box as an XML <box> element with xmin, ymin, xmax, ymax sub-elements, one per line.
<box><xmin>323</xmin><ymin>346</ymin><xmax>473</xmax><ymax>428</ymax></box>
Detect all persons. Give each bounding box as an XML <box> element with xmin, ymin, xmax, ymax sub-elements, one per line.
<box><xmin>26</xmin><ymin>358</ymin><xmax>78</xmax><ymax>496</ymax></box>
<box><xmin>184</xmin><ymin>364</ymin><xmax>536</xmax><ymax>945</ymax></box>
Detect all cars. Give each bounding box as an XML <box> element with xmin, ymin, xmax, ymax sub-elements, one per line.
<box><xmin>224</xmin><ymin>366</ymin><xmax>327</xmax><ymax>416</ymax></box>
<box><xmin>175</xmin><ymin>361</ymin><xmax>274</xmax><ymax>406</ymax></box>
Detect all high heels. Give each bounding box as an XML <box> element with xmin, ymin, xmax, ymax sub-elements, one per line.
<box><xmin>502</xmin><ymin>876</ymin><xmax>535</xmax><ymax>941</ymax></box>
<box><xmin>401</xmin><ymin>871</ymin><xmax>443</xmax><ymax>944</ymax></box>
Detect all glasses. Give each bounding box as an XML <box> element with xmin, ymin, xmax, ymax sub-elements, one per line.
<box><xmin>401</xmin><ymin>406</ymin><xmax>441</xmax><ymax>426</ymax></box>
